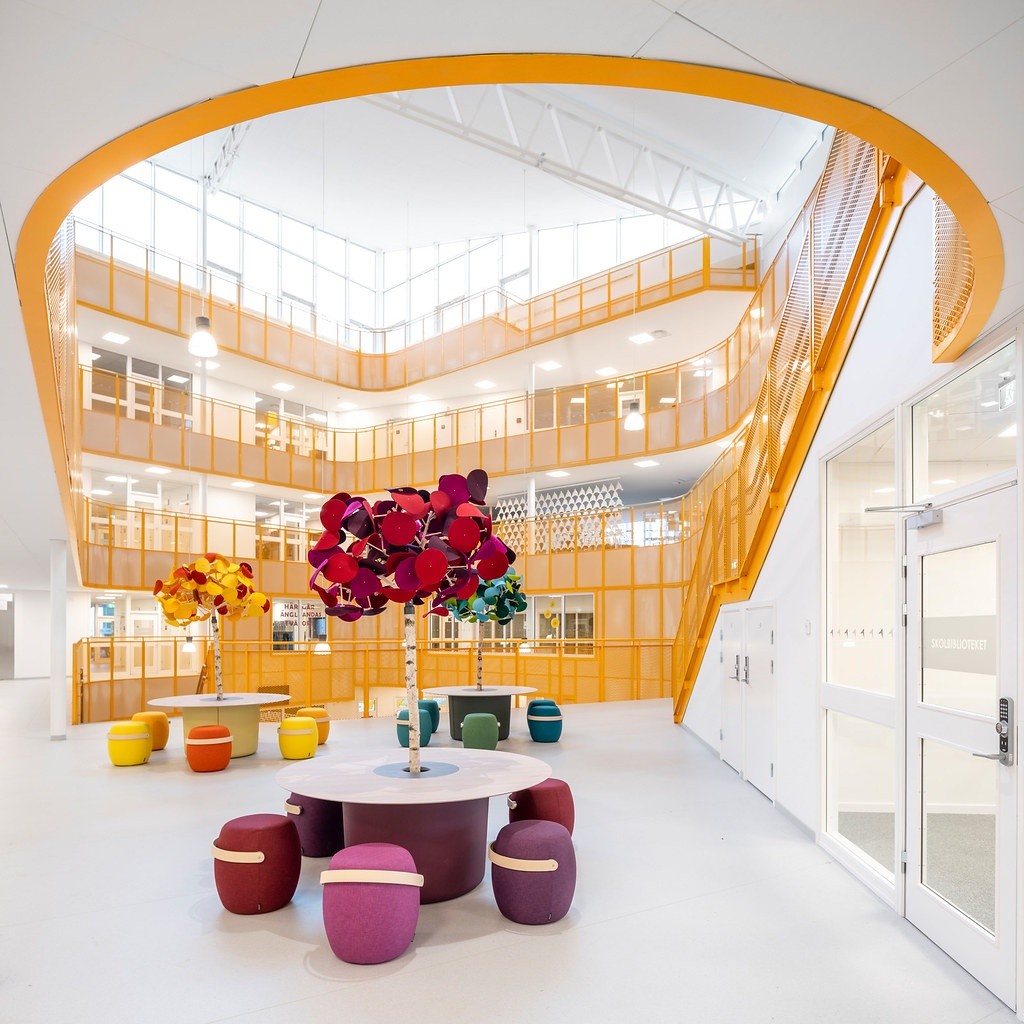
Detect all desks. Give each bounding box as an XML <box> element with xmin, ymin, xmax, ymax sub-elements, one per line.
<box><xmin>274</xmin><ymin>747</ymin><xmax>553</xmax><ymax>905</ymax></box>
<box><xmin>147</xmin><ymin>693</ymin><xmax>293</xmax><ymax>759</ymax></box>
<box><xmin>421</xmin><ymin>685</ymin><xmax>539</xmax><ymax>742</ymax></box>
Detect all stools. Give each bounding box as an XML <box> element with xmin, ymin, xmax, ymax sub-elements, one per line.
<box><xmin>529</xmin><ymin>706</ymin><xmax>563</xmax><ymax>744</ymax></box>
<box><xmin>488</xmin><ymin>820</ymin><xmax>577</xmax><ymax>926</ymax></box>
<box><xmin>418</xmin><ymin>700</ymin><xmax>441</xmax><ymax>734</ymax></box>
<box><xmin>211</xmin><ymin>814</ymin><xmax>302</xmax><ymax>915</ymax></box>
<box><xmin>396</xmin><ymin>709</ymin><xmax>432</xmax><ymax>746</ymax></box>
<box><xmin>505</xmin><ymin>778</ymin><xmax>576</xmax><ymax>838</ymax></box>
<box><xmin>282</xmin><ymin>792</ymin><xmax>344</xmax><ymax>857</ymax></box>
<box><xmin>105</xmin><ymin>721</ymin><xmax>153</xmax><ymax>767</ymax></box>
<box><xmin>188</xmin><ymin>724</ymin><xmax>234</xmax><ymax>774</ymax></box>
<box><xmin>132</xmin><ymin>711</ymin><xmax>171</xmax><ymax>751</ymax></box>
<box><xmin>527</xmin><ymin>700</ymin><xmax>555</xmax><ymax>730</ymax></box>
<box><xmin>276</xmin><ymin>717</ymin><xmax>318</xmax><ymax>759</ymax></box>
<box><xmin>320</xmin><ymin>843</ymin><xmax>424</xmax><ymax>966</ymax></box>
<box><xmin>296</xmin><ymin>707</ymin><xmax>332</xmax><ymax>745</ymax></box>
<box><xmin>460</xmin><ymin>713</ymin><xmax>501</xmax><ymax>751</ymax></box>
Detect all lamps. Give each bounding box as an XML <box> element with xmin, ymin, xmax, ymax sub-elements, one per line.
<box><xmin>182</xmin><ymin>636</ymin><xmax>196</xmax><ymax>653</ymax></box>
<box><xmin>314</xmin><ymin>634</ymin><xmax>331</xmax><ymax>656</ymax></box>
<box><xmin>624</xmin><ymin>402</ymin><xmax>645</xmax><ymax>431</ymax></box>
<box><xmin>187</xmin><ymin>179</ymin><xmax>220</xmax><ymax>357</ymax></box>
